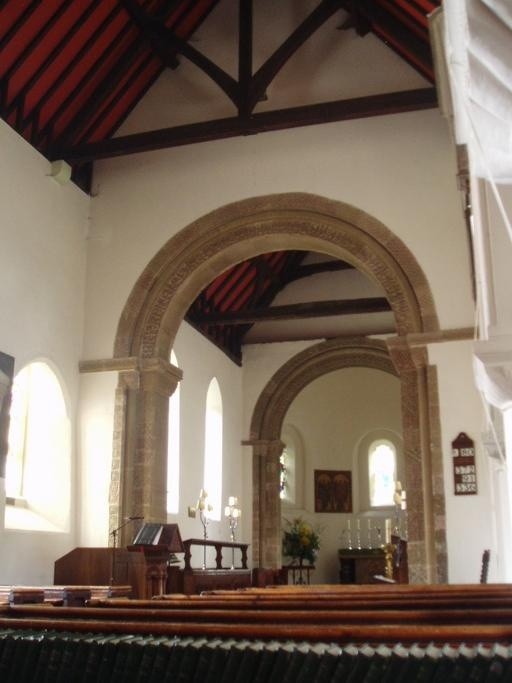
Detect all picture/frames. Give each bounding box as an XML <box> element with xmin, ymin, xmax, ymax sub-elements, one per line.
<box><xmin>314</xmin><ymin>470</ymin><xmax>352</xmax><ymax>513</ymax></box>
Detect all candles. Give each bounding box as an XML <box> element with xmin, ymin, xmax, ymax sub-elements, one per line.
<box><xmin>367</xmin><ymin>520</ymin><xmax>370</xmax><ymax>528</ymax></box>
<box><xmin>347</xmin><ymin>520</ymin><xmax>350</xmax><ymax>529</ymax></box>
<box><xmin>385</xmin><ymin>518</ymin><xmax>391</xmax><ymax>544</ymax></box>
<box><xmin>357</xmin><ymin>520</ymin><xmax>360</xmax><ymax>528</ymax></box>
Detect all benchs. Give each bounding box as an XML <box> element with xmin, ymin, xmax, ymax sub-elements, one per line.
<box><xmin>0</xmin><ymin>584</ymin><xmax>512</xmax><ymax>683</ymax></box>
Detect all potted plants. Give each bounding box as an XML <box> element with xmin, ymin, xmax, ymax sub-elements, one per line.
<box><xmin>282</xmin><ymin>513</ymin><xmax>329</xmax><ymax>565</ymax></box>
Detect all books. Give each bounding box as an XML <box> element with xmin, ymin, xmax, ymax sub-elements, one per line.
<box><xmin>132</xmin><ymin>521</ymin><xmax>165</xmax><ymax>544</ymax></box>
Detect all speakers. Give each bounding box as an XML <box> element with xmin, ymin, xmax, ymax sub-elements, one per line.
<box><xmin>50</xmin><ymin>159</ymin><xmax>73</xmax><ymax>186</ymax></box>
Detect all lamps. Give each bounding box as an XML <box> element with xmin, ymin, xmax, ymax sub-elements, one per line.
<box><xmin>196</xmin><ymin>488</ymin><xmax>213</xmax><ymax>571</ymax></box>
<box><xmin>225</xmin><ymin>496</ymin><xmax>242</xmax><ymax>569</ymax></box>
